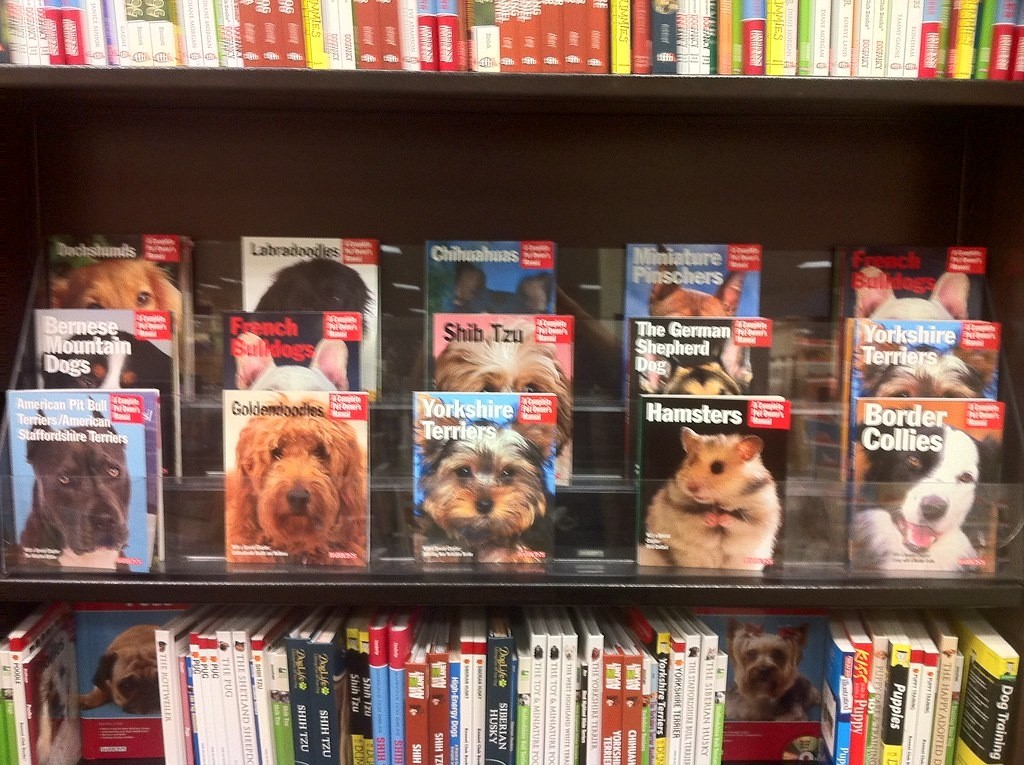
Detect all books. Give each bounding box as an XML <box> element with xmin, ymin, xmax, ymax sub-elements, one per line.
<box><xmin>0</xmin><ymin>2</ymin><xmax>1024</xmax><ymax>79</ymax></box>
<box><xmin>1</xmin><ymin>597</ymin><xmax>1024</xmax><ymax>765</ymax></box>
<box><xmin>11</xmin><ymin>230</ymin><xmax>1004</xmax><ymax>576</ymax></box>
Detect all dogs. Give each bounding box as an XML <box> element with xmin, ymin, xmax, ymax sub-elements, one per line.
<box><xmin>56</xmin><ymin>250</ymin><xmax>186</xmax><ymax>316</ymax></box>
<box><xmin>226</xmin><ymin>398</ymin><xmax>367</xmax><ymax>563</ymax></box>
<box><xmin>415</xmin><ymin>397</ymin><xmax>553</xmax><ymax>568</ymax></box>
<box><xmin>432</xmin><ymin>322</ymin><xmax>568</xmax><ymax>446</ymax></box>
<box><xmin>725</xmin><ymin>613</ymin><xmax>819</xmax><ymax>721</ymax></box>
<box><xmin>850</xmin><ymin>418</ymin><xmax>999</xmax><ymax>573</ymax></box>
<box><xmin>79</xmin><ymin>626</ymin><xmax>164</xmax><ymax>718</ymax></box>
<box><xmin>15</xmin><ymin>395</ymin><xmax>130</xmax><ymax>567</ymax></box>
<box><xmin>857</xmin><ymin>320</ymin><xmax>998</xmax><ymax>401</ymax></box>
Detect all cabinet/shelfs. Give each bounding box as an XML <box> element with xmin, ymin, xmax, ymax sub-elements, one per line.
<box><xmin>1</xmin><ymin>69</ymin><xmax>1024</xmax><ymax>614</ymax></box>
